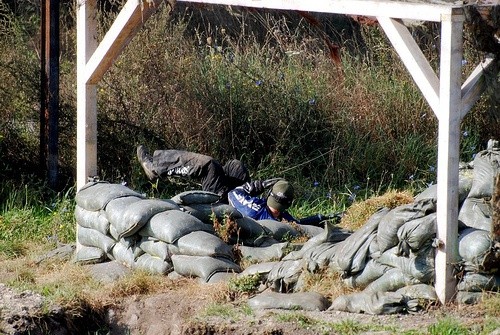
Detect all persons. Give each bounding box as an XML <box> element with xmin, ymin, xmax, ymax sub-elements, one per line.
<box><xmin>136</xmin><ymin>144</ymin><xmax>294</xmax><ymax>222</ymax></box>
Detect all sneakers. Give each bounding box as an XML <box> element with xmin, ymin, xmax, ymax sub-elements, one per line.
<box><xmin>137</xmin><ymin>145</ymin><xmax>157</xmax><ymax>180</ymax></box>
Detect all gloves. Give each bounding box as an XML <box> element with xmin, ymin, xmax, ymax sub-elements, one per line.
<box><xmin>263</xmin><ymin>178</ymin><xmax>284</xmax><ymax>188</ymax></box>
<box><xmin>320</xmin><ymin>215</ymin><xmax>341</xmax><ymax>225</ymax></box>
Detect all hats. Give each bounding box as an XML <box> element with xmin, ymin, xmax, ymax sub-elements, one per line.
<box><xmin>266</xmin><ymin>179</ymin><xmax>294</xmax><ymax>212</ymax></box>
